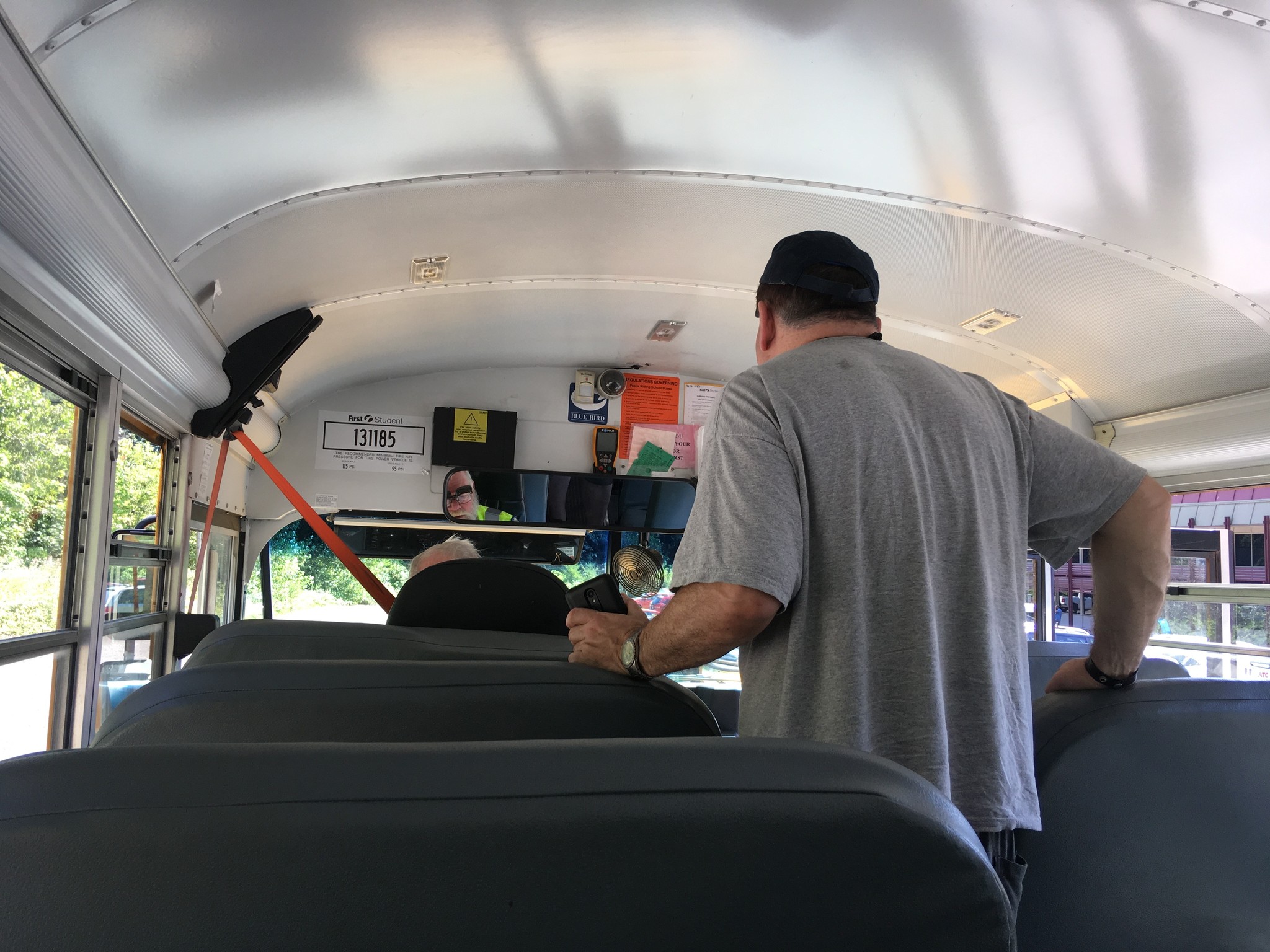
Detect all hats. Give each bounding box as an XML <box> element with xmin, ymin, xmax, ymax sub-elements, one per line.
<box><xmin>756</xmin><ymin>230</ymin><xmax>880</xmax><ymax>319</ymax></box>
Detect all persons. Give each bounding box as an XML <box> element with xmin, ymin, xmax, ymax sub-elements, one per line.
<box><xmin>1054</xmin><ymin>604</ymin><xmax>1062</xmax><ymax>625</ymax></box>
<box><xmin>446</xmin><ymin>470</ymin><xmax>520</xmax><ymax>522</ymax></box>
<box><xmin>407</xmin><ymin>533</ymin><xmax>481</xmax><ymax>578</ymax></box>
<box><xmin>565</xmin><ymin>230</ymin><xmax>1173</xmax><ymax>933</ymax></box>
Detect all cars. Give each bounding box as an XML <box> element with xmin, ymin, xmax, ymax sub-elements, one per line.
<box><xmin>649</xmin><ymin>595</ymin><xmax>674</xmax><ymax>613</ymax></box>
<box><xmin>104</xmin><ymin>577</ymin><xmax>147</xmax><ymax>621</ymax></box>
<box><xmin>631</xmin><ymin>594</ymin><xmax>669</xmax><ymax>609</ymax></box>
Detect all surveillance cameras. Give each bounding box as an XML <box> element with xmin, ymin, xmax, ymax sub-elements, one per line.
<box><xmin>596</xmin><ymin>368</ymin><xmax>627</xmax><ymax>398</ymax></box>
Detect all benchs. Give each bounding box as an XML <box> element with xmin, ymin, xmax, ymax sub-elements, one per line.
<box><xmin>0</xmin><ymin>621</ymin><xmax>1269</xmax><ymax>951</ymax></box>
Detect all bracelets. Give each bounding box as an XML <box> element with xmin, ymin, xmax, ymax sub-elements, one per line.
<box><xmin>1084</xmin><ymin>654</ymin><xmax>1139</xmax><ymax>689</ymax></box>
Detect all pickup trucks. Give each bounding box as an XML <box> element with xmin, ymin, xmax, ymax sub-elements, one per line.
<box><xmin>1053</xmin><ymin>590</ymin><xmax>1094</xmax><ymax>613</ymax></box>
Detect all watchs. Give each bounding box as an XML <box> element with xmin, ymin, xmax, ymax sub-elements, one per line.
<box><xmin>619</xmin><ymin>627</ymin><xmax>661</xmax><ymax>680</ymax></box>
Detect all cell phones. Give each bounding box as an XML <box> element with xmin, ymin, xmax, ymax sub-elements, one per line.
<box><xmin>565</xmin><ymin>574</ymin><xmax>627</xmax><ymax>615</ymax></box>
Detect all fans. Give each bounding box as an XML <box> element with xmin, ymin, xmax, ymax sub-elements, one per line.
<box><xmin>612</xmin><ymin>545</ymin><xmax>665</xmax><ymax>598</ymax></box>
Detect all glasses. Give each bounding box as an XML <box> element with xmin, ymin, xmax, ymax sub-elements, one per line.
<box><xmin>446</xmin><ymin>484</ymin><xmax>473</xmax><ymax>508</ymax></box>
